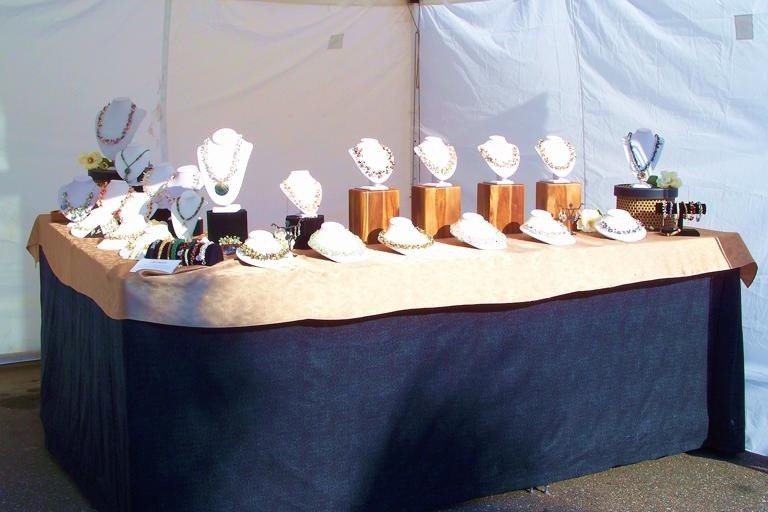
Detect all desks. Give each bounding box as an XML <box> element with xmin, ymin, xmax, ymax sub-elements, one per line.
<box><xmin>30</xmin><ymin>213</ymin><xmax>746</xmax><ymax>512</ymax></box>
